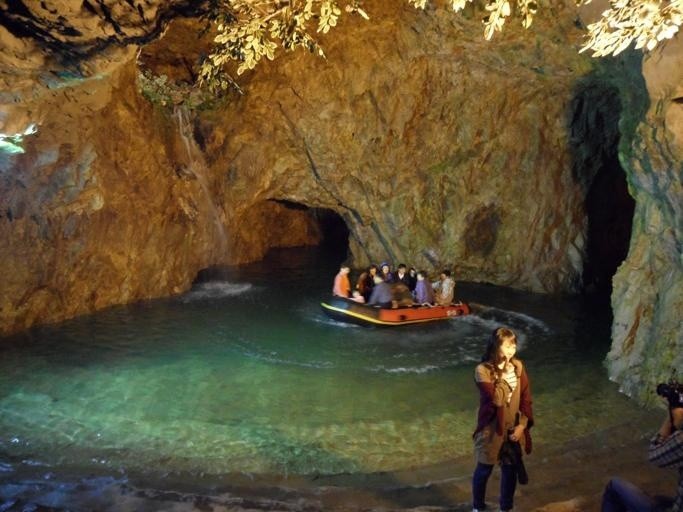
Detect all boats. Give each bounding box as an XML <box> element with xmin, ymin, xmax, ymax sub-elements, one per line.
<box><xmin>316</xmin><ymin>286</ymin><xmax>473</xmax><ymax>331</ymax></box>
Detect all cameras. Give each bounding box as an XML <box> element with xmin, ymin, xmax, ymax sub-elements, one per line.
<box><xmin>657</xmin><ymin>377</ymin><xmax>683</xmax><ymax>408</ymax></box>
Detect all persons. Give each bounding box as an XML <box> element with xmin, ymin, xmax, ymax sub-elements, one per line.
<box><xmin>472</xmin><ymin>327</ymin><xmax>534</xmax><ymax>512</ymax></box>
<box><xmin>333</xmin><ymin>263</ymin><xmax>456</xmax><ymax>310</ymax></box>
<box><xmin>601</xmin><ymin>395</ymin><xmax>683</xmax><ymax>512</ymax></box>
